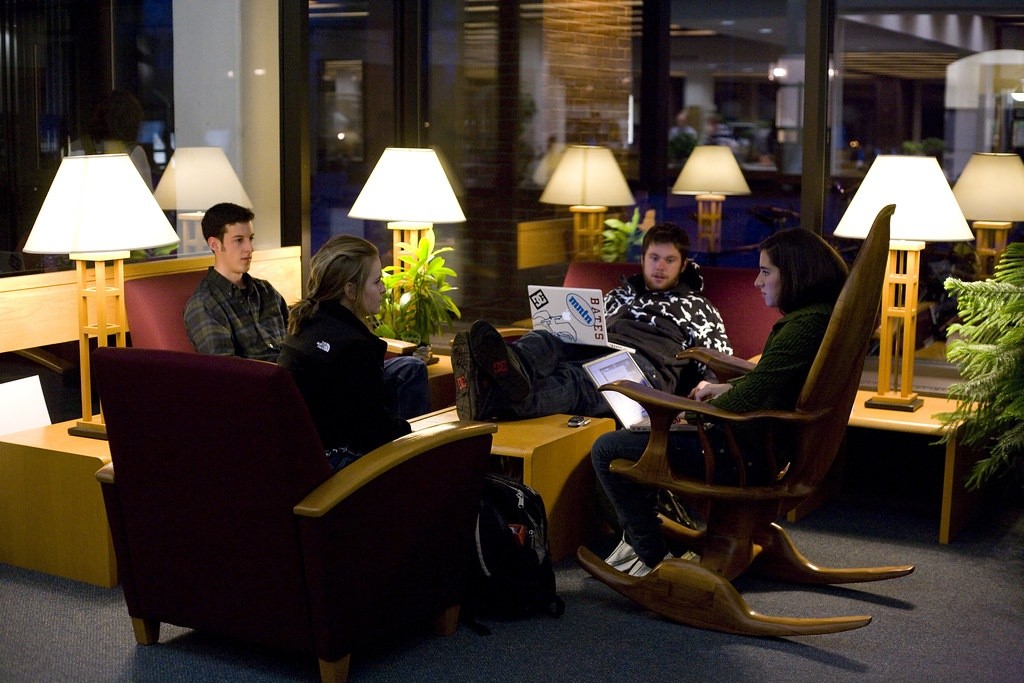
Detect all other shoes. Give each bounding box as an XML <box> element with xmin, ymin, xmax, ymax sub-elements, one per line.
<box><xmin>451</xmin><ymin>331</ymin><xmax>502</xmax><ymax>424</ymax></box>
<box><xmin>470</xmin><ymin>320</ymin><xmax>532</xmax><ymax>401</ymax></box>
<box><xmin>602</xmin><ymin>531</ymin><xmax>639</xmax><ymax>572</ymax></box>
<box><xmin>628</xmin><ymin>553</ymin><xmax>673</xmax><ymax>576</ymax></box>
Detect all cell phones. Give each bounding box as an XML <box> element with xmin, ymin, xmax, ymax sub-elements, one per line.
<box><xmin>567</xmin><ymin>416</ymin><xmax>585</xmax><ymax>426</ymax></box>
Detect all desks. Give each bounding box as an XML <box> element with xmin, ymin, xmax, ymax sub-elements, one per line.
<box><xmin>0</xmin><ymin>415</ymin><xmax>116</xmax><ymax>589</ymax></box>
<box><xmin>848</xmin><ymin>391</ymin><xmax>987</xmax><ymax>545</ymax></box>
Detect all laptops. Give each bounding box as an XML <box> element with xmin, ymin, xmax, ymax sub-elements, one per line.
<box><xmin>582</xmin><ymin>349</ymin><xmax>715</xmax><ymax>431</ymax></box>
<box><xmin>528</xmin><ymin>285</ymin><xmax>636</xmax><ymax>354</ymax></box>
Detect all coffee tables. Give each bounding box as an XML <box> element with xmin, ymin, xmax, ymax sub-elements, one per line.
<box><xmin>405</xmin><ymin>405</ymin><xmax>616</xmax><ymax>558</ymax></box>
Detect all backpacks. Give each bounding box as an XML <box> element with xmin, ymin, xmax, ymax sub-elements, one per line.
<box><xmin>474</xmin><ymin>474</ymin><xmax>567</xmax><ymax>619</ymax></box>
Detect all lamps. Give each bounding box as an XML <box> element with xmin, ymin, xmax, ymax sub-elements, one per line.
<box><xmin>538</xmin><ymin>145</ymin><xmax>638</xmax><ymax>260</ymax></box>
<box><xmin>347</xmin><ymin>149</ymin><xmax>467</xmax><ymax>363</ymax></box>
<box><xmin>672</xmin><ymin>144</ymin><xmax>752</xmax><ymax>247</ymax></box>
<box><xmin>23</xmin><ymin>155</ymin><xmax>180</xmax><ymax>441</ymax></box>
<box><xmin>833</xmin><ymin>154</ymin><xmax>975</xmax><ymax>410</ymax></box>
<box><xmin>952</xmin><ymin>151</ymin><xmax>1024</xmax><ymax>280</ymax></box>
<box><xmin>151</xmin><ymin>148</ymin><xmax>254</xmax><ymax>253</ymax></box>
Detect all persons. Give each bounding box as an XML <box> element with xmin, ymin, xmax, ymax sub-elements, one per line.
<box><xmin>606</xmin><ymin>229</ymin><xmax>851</xmax><ymax>573</ymax></box>
<box><xmin>450</xmin><ymin>222</ymin><xmax>732</xmax><ymax>423</ymax></box>
<box><xmin>668</xmin><ymin>111</ymin><xmax>746</xmax><ymax>168</ymax></box>
<box><xmin>183</xmin><ymin>202</ymin><xmax>428</xmax><ymax>417</ymax></box>
<box><xmin>279</xmin><ymin>235</ymin><xmax>565</xmax><ymax>617</ymax></box>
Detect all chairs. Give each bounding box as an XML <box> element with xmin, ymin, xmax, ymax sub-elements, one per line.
<box><xmin>577</xmin><ymin>205</ymin><xmax>914</xmax><ymax>637</ymax></box>
<box><xmin>498</xmin><ymin>262</ymin><xmax>787</xmax><ymax>366</ymax></box>
<box><xmin>121</xmin><ymin>270</ymin><xmax>418</xmax><ymax>359</ymax></box>
<box><xmin>88</xmin><ymin>345</ymin><xmax>497</xmax><ymax>683</ymax></box>
